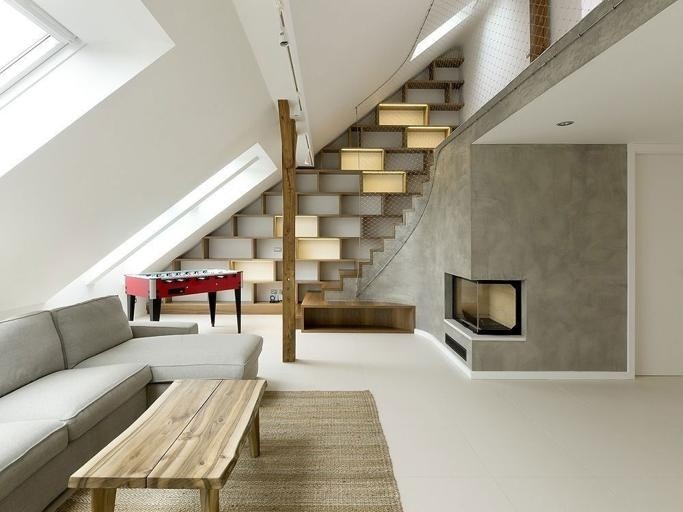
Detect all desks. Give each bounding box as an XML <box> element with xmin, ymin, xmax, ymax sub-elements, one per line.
<box><xmin>124</xmin><ymin>269</ymin><xmax>245</xmax><ymax>336</ymax></box>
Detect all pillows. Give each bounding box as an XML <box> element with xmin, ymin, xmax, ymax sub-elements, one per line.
<box><xmin>49</xmin><ymin>293</ymin><xmax>134</xmax><ymax>369</ymax></box>
<box><xmin>0</xmin><ymin>310</ymin><xmax>67</xmax><ymax>399</ymax></box>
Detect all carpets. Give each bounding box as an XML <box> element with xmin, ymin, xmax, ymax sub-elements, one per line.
<box><xmin>53</xmin><ymin>390</ymin><xmax>404</xmax><ymax>512</ymax></box>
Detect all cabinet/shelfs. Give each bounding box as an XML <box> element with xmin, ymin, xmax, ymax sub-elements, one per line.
<box><xmin>146</xmin><ymin>55</ymin><xmax>466</xmax><ymax>334</ymax></box>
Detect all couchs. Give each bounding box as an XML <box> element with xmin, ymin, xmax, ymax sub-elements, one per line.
<box><xmin>0</xmin><ymin>294</ymin><xmax>264</xmax><ymax>512</ymax></box>
